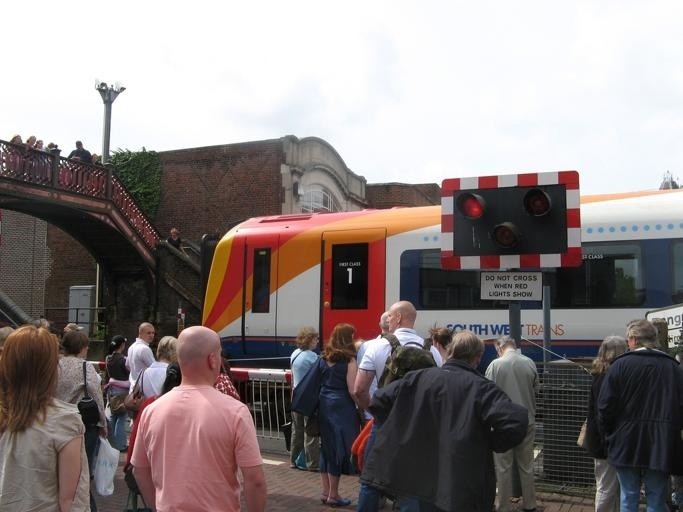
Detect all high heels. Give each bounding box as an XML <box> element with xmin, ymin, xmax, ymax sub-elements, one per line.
<box><xmin>327</xmin><ymin>497</ymin><xmax>350</xmax><ymax>508</ymax></box>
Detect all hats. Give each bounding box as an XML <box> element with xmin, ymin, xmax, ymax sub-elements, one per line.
<box><xmin>111</xmin><ymin>335</ymin><xmax>127</xmax><ymax>346</ymax></box>
<box><xmin>66</xmin><ymin>323</ymin><xmax>84</xmax><ymax>331</ymax></box>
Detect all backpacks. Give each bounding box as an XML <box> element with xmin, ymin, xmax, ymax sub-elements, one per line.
<box><xmin>378</xmin><ymin>334</ymin><xmax>437</xmax><ymax>388</ymax></box>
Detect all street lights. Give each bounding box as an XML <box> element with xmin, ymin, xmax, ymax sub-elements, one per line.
<box><xmin>94</xmin><ymin>82</ymin><xmax>127</xmax><ymax>332</ymax></box>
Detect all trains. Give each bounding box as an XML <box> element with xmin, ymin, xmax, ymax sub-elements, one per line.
<box><xmin>198</xmin><ymin>187</ymin><xmax>682</xmax><ymax>451</ymax></box>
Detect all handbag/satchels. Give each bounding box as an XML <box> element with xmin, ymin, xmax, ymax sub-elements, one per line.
<box><xmin>77</xmin><ymin>396</ymin><xmax>99</xmax><ymax>438</ymax></box>
<box><xmin>577</xmin><ymin>418</ymin><xmax>587</xmax><ymax>448</ymax></box>
<box><xmin>289</xmin><ymin>356</ymin><xmax>321</xmax><ymax>436</ymax></box>
<box><xmin>110</xmin><ymin>368</ymin><xmax>145</xmax><ymax>414</ymax></box>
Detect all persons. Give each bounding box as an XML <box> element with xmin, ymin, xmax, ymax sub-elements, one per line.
<box><xmin>584</xmin><ymin>319</ymin><xmax>683</xmax><ymax>511</ymax></box>
<box><xmin>352</xmin><ymin>299</ymin><xmax>444</xmax><ymax>510</ymax></box>
<box><xmin>2</xmin><ymin>129</ymin><xmax>98</xmax><ymax>192</ymax></box>
<box><xmin>431</xmin><ymin>327</ymin><xmax>538</xmax><ymax>510</ymax></box>
<box><xmin>0</xmin><ymin>318</ymin><xmax>266</xmax><ymax>512</ymax></box>
<box><xmin>163</xmin><ymin>228</ymin><xmax>183</xmax><ymax>253</ymax></box>
<box><xmin>356</xmin><ymin>331</ymin><xmax>530</xmax><ymax>510</ymax></box>
<box><xmin>290</xmin><ymin>311</ymin><xmax>389</xmax><ymax>507</ymax></box>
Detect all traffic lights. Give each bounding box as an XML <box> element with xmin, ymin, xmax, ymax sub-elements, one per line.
<box><xmin>440</xmin><ymin>172</ymin><xmax>581</xmax><ymax>273</ymax></box>
